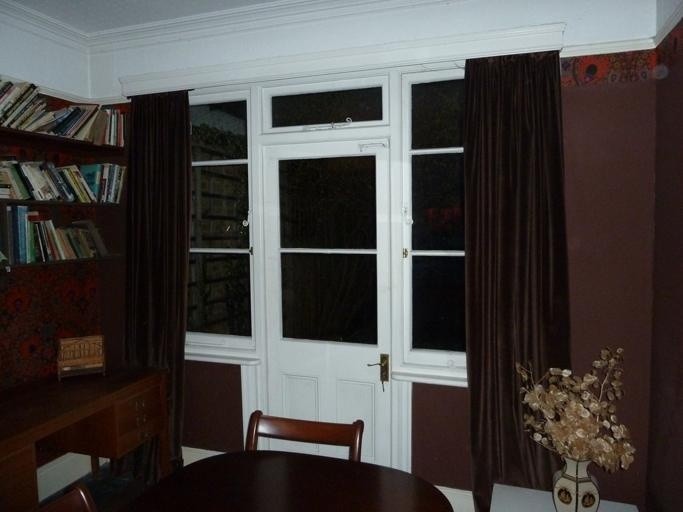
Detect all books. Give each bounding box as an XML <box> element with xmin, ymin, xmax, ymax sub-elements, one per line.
<box><xmin>185</xmin><ymin>122</ymin><xmax>249</xmax><ymax>337</ymax></box>
<box><xmin>0</xmin><ymin>79</ymin><xmax>130</xmax><ymax>266</ymax></box>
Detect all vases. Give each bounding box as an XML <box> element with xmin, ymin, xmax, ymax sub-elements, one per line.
<box><xmin>550</xmin><ymin>454</ymin><xmax>601</xmax><ymax>511</ymax></box>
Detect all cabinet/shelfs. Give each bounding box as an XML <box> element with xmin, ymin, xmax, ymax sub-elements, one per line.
<box><xmin>0</xmin><ymin>78</ymin><xmax>133</xmax><ymax>384</ymax></box>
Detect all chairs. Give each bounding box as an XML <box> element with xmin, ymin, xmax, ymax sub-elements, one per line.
<box><xmin>36</xmin><ymin>485</ymin><xmax>96</xmax><ymax>512</ymax></box>
<box><xmin>246</xmin><ymin>407</ymin><xmax>367</xmax><ymax>462</ymax></box>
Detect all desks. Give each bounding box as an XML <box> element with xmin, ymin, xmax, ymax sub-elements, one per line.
<box><xmin>107</xmin><ymin>443</ymin><xmax>459</xmax><ymax>511</ymax></box>
<box><xmin>0</xmin><ymin>361</ymin><xmax>172</xmax><ymax>511</ymax></box>
<box><xmin>486</xmin><ymin>482</ymin><xmax>646</xmax><ymax>511</ymax></box>
